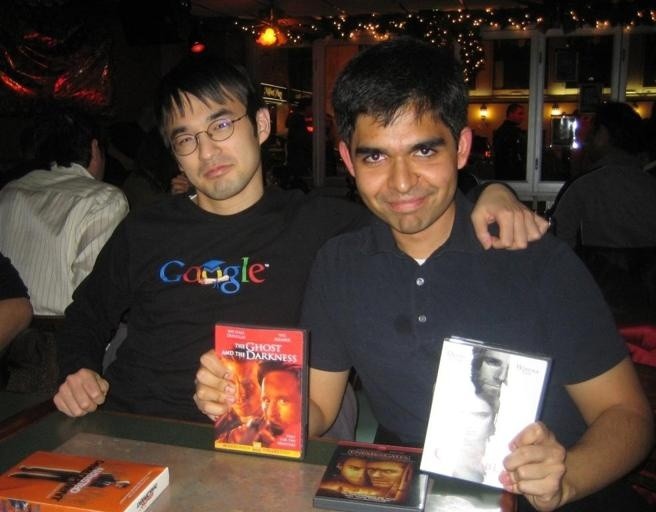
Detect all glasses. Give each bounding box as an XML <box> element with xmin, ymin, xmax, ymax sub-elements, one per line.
<box><xmin>172</xmin><ymin>114</ymin><xmax>246</xmax><ymax>156</ymax></box>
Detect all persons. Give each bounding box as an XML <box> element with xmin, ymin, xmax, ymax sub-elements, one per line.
<box><xmin>435</xmin><ymin>347</ymin><xmax>518</xmax><ymax>484</ymax></box>
<box><xmin>281</xmin><ymin>106</ymin><xmax>314</xmax><ymax>180</ymax></box>
<box><xmin>6</xmin><ymin>465</ymin><xmax>131</xmax><ymax>492</ymax></box>
<box><xmin>492</xmin><ymin>104</ymin><xmax>527</xmax><ymax>182</ymax></box>
<box><xmin>216</xmin><ymin>347</ymin><xmax>301</xmax><ymax>455</ymax></box>
<box><xmin>193</xmin><ymin>37</ymin><xmax>656</xmax><ymax>512</ymax></box>
<box><xmin>552</xmin><ymin>101</ymin><xmax>656</xmax><ymax>323</ymax></box>
<box><xmin>0</xmin><ymin>104</ymin><xmax>131</xmax><ymax>367</ymax></box>
<box><xmin>51</xmin><ymin>57</ymin><xmax>547</xmax><ymax>419</ymax></box>
<box><xmin>316</xmin><ymin>450</ymin><xmax>409</xmax><ymax>501</ymax></box>
<box><xmin>0</xmin><ymin>253</ymin><xmax>36</xmax><ymax>350</ymax></box>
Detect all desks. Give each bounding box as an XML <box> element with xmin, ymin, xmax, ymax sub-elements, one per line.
<box><xmin>0</xmin><ymin>404</ymin><xmax>520</xmax><ymax>512</ymax></box>
